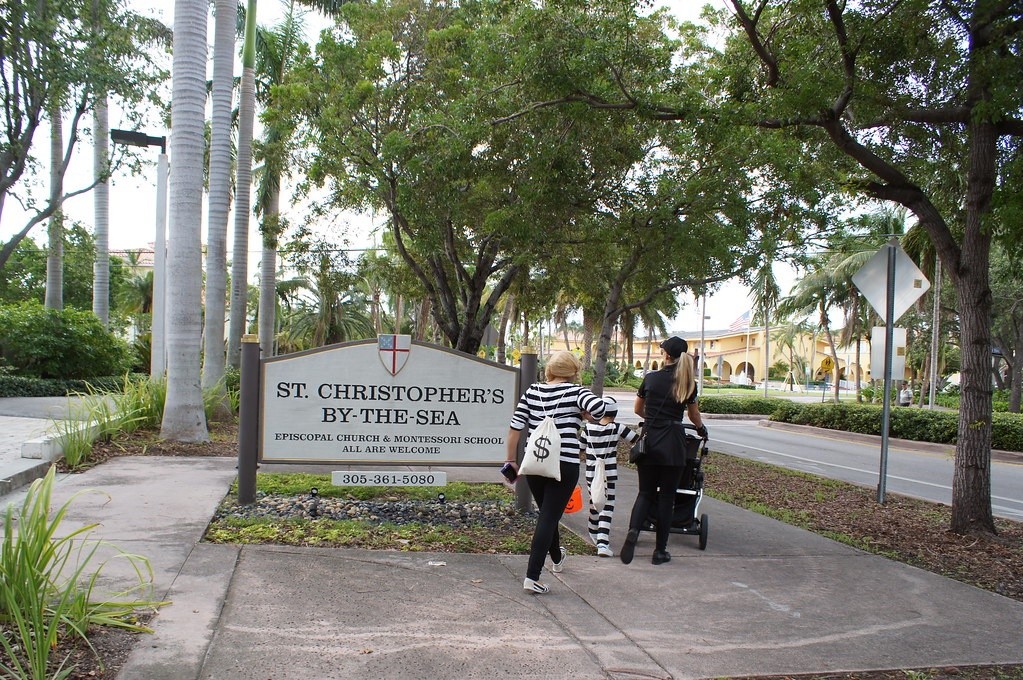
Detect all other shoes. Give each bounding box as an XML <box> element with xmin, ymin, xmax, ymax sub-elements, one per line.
<box><xmin>652</xmin><ymin>550</ymin><xmax>671</xmax><ymax>565</ymax></box>
<box><xmin>596</xmin><ymin>543</ymin><xmax>613</xmax><ymax>557</ymax></box>
<box><xmin>620</xmin><ymin>528</ymin><xmax>639</xmax><ymax>564</ymax></box>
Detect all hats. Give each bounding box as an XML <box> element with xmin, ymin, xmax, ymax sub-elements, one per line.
<box><xmin>600</xmin><ymin>396</ymin><xmax>618</xmax><ymax>413</ymax></box>
<box><xmin>902</xmin><ymin>380</ymin><xmax>908</xmax><ymax>385</ymax></box>
<box><xmin>660</xmin><ymin>336</ymin><xmax>688</xmax><ymax>358</ymax></box>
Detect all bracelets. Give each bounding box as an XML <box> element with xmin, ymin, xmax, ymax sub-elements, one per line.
<box><xmin>503</xmin><ymin>459</ymin><xmax>516</xmax><ymax>463</ymax></box>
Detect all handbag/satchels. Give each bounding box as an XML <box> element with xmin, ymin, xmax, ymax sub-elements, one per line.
<box><xmin>629</xmin><ymin>433</ymin><xmax>649</xmax><ymax>467</ymax></box>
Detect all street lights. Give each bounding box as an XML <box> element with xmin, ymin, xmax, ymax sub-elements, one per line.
<box><xmin>110</xmin><ymin>129</ymin><xmax>168</xmax><ymax>376</ymax></box>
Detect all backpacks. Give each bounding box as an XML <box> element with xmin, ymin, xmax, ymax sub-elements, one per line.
<box><xmin>588</xmin><ymin>422</ymin><xmax>614</xmax><ymax>514</ymax></box>
<box><xmin>516</xmin><ymin>382</ymin><xmax>575</xmax><ymax>482</ymax></box>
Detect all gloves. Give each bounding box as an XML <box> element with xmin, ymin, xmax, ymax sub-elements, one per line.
<box><xmin>696</xmin><ymin>424</ymin><xmax>708</xmax><ymax>437</ymax></box>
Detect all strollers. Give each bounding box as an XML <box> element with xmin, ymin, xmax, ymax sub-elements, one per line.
<box><xmin>638</xmin><ymin>422</ymin><xmax>709</xmax><ymax>550</ymax></box>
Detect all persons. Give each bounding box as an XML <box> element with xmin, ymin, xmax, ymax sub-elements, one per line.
<box><xmin>578</xmin><ymin>395</ymin><xmax>640</xmax><ymax>557</ymax></box>
<box><xmin>619</xmin><ymin>335</ymin><xmax>708</xmax><ymax>566</ymax></box>
<box><xmin>501</xmin><ymin>351</ymin><xmax>606</xmax><ymax>594</ymax></box>
<box><xmin>899</xmin><ymin>380</ymin><xmax>913</xmax><ymax>407</ymax></box>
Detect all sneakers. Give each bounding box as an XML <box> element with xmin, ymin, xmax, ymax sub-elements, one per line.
<box><xmin>523</xmin><ymin>578</ymin><xmax>550</xmax><ymax>594</ymax></box>
<box><xmin>553</xmin><ymin>546</ymin><xmax>566</xmax><ymax>572</ymax></box>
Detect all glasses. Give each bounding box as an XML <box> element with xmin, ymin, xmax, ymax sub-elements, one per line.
<box><xmin>660</xmin><ymin>350</ymin><xmax>666</xmax><ymax>355</ymax></box>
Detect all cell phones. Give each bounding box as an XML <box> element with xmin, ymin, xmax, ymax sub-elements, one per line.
<box><xmin>500</xmin><ymin>463</ymin><xmax>520</xmax><ymax>484</ymax></box>
